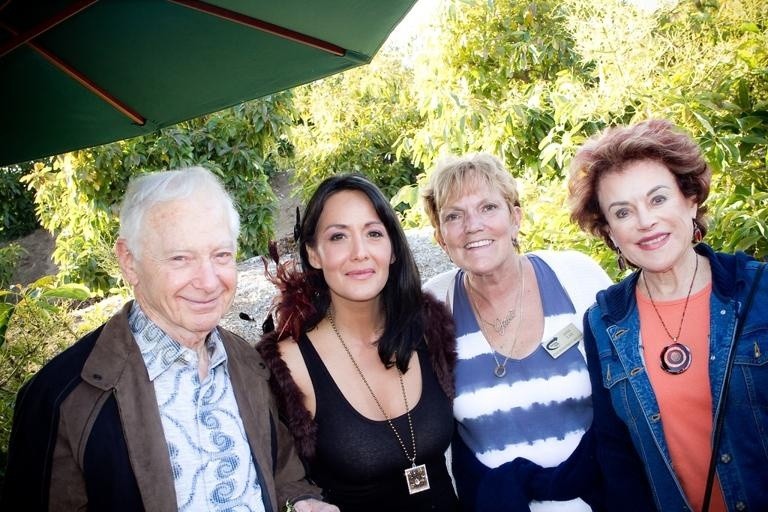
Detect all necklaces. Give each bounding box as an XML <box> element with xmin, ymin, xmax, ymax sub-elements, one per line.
<box><xmin>641</xmin><ymin>252</ymin><xmax>700</xmax><ymax>376</ymax></box>
<box><xmin>324</xmin><ymin>310</ymin><xmax>430</xmax><ymax>498</ymax></box>
<box><xmin>466</xmin><ymin>279</ymin><xmax>520</xmax><ymax>337</ymax></box>
<box><xmin>468</xmin><ymin>258</ymin><xmax>530</xmax><ymax>380</ymax></box>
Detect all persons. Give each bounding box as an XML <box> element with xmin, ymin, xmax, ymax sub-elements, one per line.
<box><xmin>245</xmin><ymin>170</ymin><xmax>464</xmax><ymax>512</ymax></box>
<box><xmin>413</xmin><ymin>154</ymin><xmax>624</xmax><ymax>510</ymax></box>
<box><xmin>2</xmin><ymin>165</ymin><xmax>340</xmax><ymax>512</ymax></box>
<box><xmin>559</xmin><ymin>117</ymin><xmax>767</xmax><ymax>510</ymax></box>
